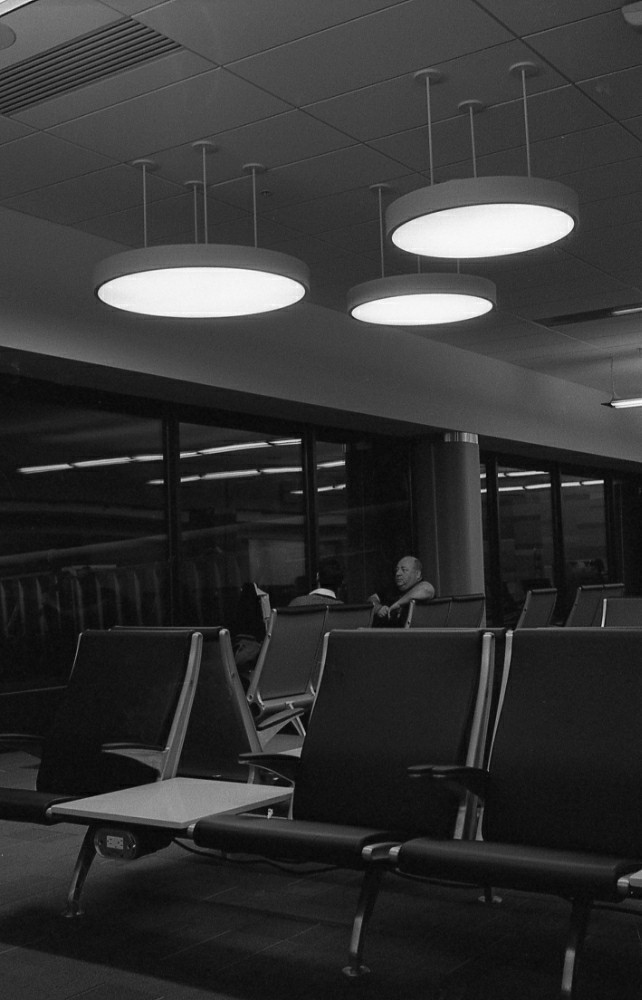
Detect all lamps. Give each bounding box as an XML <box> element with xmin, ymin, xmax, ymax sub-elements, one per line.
<box><xmin>347</xmin><ymin>181</ymin><xmax>496</xmax><ymax>326</ymax></box>
<box><xmin>380</xmin><ymin>61</ymin><xmax>580</xmax><ymax>259</ymax></box>
<box><xmin>91</xmin><ymin>137</ymin><xmax>307</xmax><ymax>319</ymax></box>
<box><xmin>600</xmin><ymin>349</ymin><xmax>642</xmax><ymax>409</ymax></box>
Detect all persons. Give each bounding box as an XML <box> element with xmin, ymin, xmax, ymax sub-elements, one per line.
<box><xmin>287</xmin><ymin>559</ymin><xmax>347</xmax><ymax>607</ymax></box>
<box><xmin>365</xmin><ymin>555</ymin><xmax>436</xmax><ymax>618</ymax></box>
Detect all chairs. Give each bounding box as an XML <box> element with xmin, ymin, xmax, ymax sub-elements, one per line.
<box><xmin>0</xmin><ymin>582</ymin><xmax>642</xmax><ymax>1000</ymax></box>
